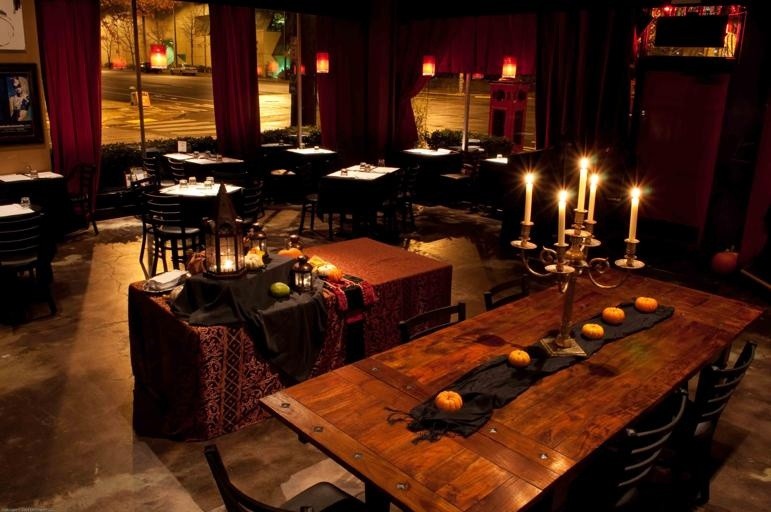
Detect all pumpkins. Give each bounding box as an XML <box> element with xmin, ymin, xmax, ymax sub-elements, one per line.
<box><xmin>581</xmin><ymin>323</ymin><xmax>605</xmax><ymax>340</ymax></box>
<box><xmin>712</xmin><ymin>250</ymin><xmax>737</xmax><ymax>273</ymax></box>
<box><xmin>508</xmin><ymin>349</ymin><xmax>531</xmax><ymax>368</ymax></box>
<box><xmin>433</xmin><ymin>390</ymin><xmax>463</xmax><ymax>412</ymax></box>
<box><xmin>634</xmin><ymin>296</ymin><xmax>658</xmax><ymax>313</ymax></box>
<box><xmin>277</xmin><ymin>248</ymin><xmax>302</xmax><ymax>260</ymax></box>
<box><xmin>317</xmin><ymin>264</ymin><xmax>341</xmax><ymax>281</ymax></box>
<box><xmin>602</xmin><ymin>306</ymin><xmax>626</xmax><ymax>325</ymax></box>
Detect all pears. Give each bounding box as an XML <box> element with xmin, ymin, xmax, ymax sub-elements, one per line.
<box><xmin>270</xmin><ymin>282</ymin><xmax>291</xmax><ymax>298</ymax></box>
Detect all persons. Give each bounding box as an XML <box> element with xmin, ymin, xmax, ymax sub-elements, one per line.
<box><xmin>7</xmin><ymin>77</ymin><xmax>32</xmax><ymax>123</ymax></box>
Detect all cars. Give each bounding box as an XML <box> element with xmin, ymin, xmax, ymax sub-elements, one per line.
<box><xmin>139</xmin><ymin>62</ymin><xmax>162</xmax><ymax>74</ymax></box>
<box><xmin>170</xmin><ymin>63</ymin><xmax>197</xmax><ymax>75</ymax></box>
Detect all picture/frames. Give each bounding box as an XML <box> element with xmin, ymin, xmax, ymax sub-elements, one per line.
<box><xmin>0</xmin><ymin>61</ymin><xmax>46</xmax><ymax>148</ymax></box>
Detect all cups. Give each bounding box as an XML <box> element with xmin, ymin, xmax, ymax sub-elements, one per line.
<box><xmin>341</xmin><ymin>169</ymin><xmax>348</xmax><ymax>177</ymax></box>
<box><xmin>205</xmin><ymin>151</ymin><xmax>211</xmax><ymax>159</ymax></box>
<box><xmin>360</xmin><ymin>162</ymin><xmax>371</xmax><ymax>173</ymax></box>
<box><xmin>378</xmin><ymin>160</ymin><xmax>386</xmax><ymax>169</ymax></box>
<box><xmin>189</xmin><ymin>176</ymin><xmax>197</xmax><ymax>186</ymax></box>
<box><xmin>204</xmin><ymin>177</ymin><xmax>215</xmax><ymax>190</ymax></box>
<box><xmin>24</xmin><ymin>166</ymin><xmax>39</xmax><ymax>179</ymax></box>
<box><xmin>315</xmin><ymin>146</ymin><xmax>320</xmax><ymax>152</ymax></box>
<box><xmin>217</xmin><ymin>155</ymin><xmax>223</xmax><ymax>163</ymax></box>
<box><xmin>279</xmin><ymin>140</ymin><xmax>284</xmax><ymax>146</ymax></box>
<box><xmin>180</xmin><ymin>179</ymin><xmax>188</xmax><ymax>189</ymax></box>
<box><xmin>300</xmin><ymin>143</ymin><xmax>306</xmax><ymax>150</ymax></box>
<box><xmin>497</xmin><ymin>154</ymin><xmax>503</xmax><ymax>161</ymax></box>
<box><xmin>21</xmin><ymin>197</ymin><xmax>32</xmax><ymax>212</ymax></box>
<box><xmin>194</xmin><ymin>151</ymin><xmax>200</xmax><ymax>160</ymax></box>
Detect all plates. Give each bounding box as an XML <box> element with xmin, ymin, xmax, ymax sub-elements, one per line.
<box><xmin>143</xmin><ymin>281</ymin><xmax>179</xmax><ymax>293</ymax></box>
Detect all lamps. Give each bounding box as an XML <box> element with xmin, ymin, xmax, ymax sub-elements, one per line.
<box><xmin>308</xmin><ymin>48</ymin><xmax>332</xmax><ymax>79</ymax></box>
<box><xmin>417</xmin><ymin>52</ymin><xmax>437</xmax><ymax>79</ymax></box>
<box><xmin>500</xmin><ymin>51</ymin><xmax>519</xmax><ymax>80</ymax></box>
<box><xmin>146</xmin><ymin>35</ymin><xmax>172</xmax><ymax>73</ymax></box>
